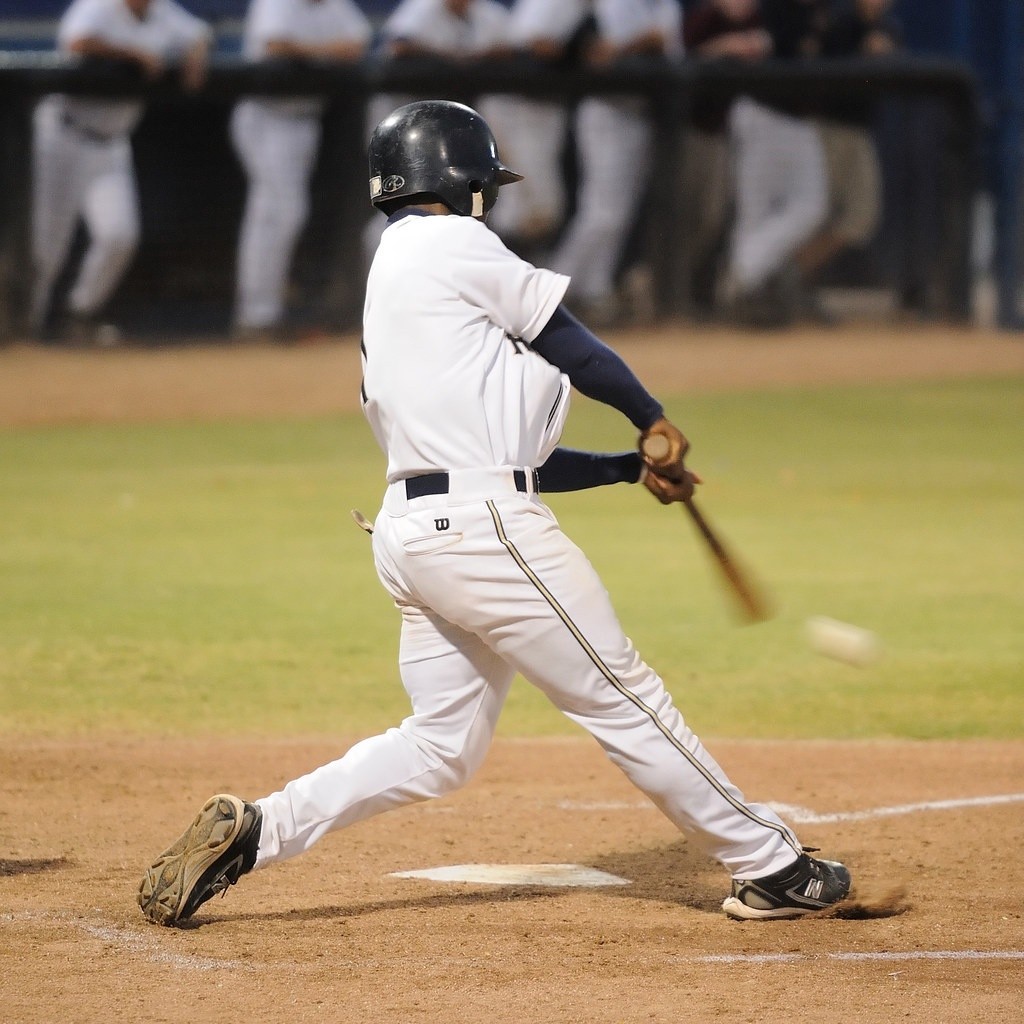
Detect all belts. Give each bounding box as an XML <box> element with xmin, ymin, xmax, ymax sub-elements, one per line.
<box><xmin>405</xmin><ymin>469</ymin><xmax>540</xmax><ymax>500</ymax></box>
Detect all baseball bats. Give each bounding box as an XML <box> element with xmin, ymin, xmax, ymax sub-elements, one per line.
<box><xmin>640</xmin><ymin>433</ymin><xmax>778</xmax><ymax>626</ymax></box>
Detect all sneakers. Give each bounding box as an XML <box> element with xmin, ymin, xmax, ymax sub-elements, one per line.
<box><xmin>137</xmin><ymin>794</ymin><xmax>263</xmax><ymax>925</ymax></box>
<box><xmin>723</xmin><ymin>846</ymin><xmax>851</xmax><ymax>921</ymax></box>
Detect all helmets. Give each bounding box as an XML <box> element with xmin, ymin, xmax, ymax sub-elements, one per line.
<box><xmin>369</xmin><ymin>100</ymin><xmax>525</xmax><ymax>217</ymax></box>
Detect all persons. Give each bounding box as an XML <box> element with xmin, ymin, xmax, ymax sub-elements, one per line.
<box><xmin>0</xmin><ymin>0</ymin><xmax>1024</xmax><ymax>346</ymax></box>
<box><xmin>137</xmin><ymin>99</ymin><xmax>851</xmax><ymax>927</ymax></box>
